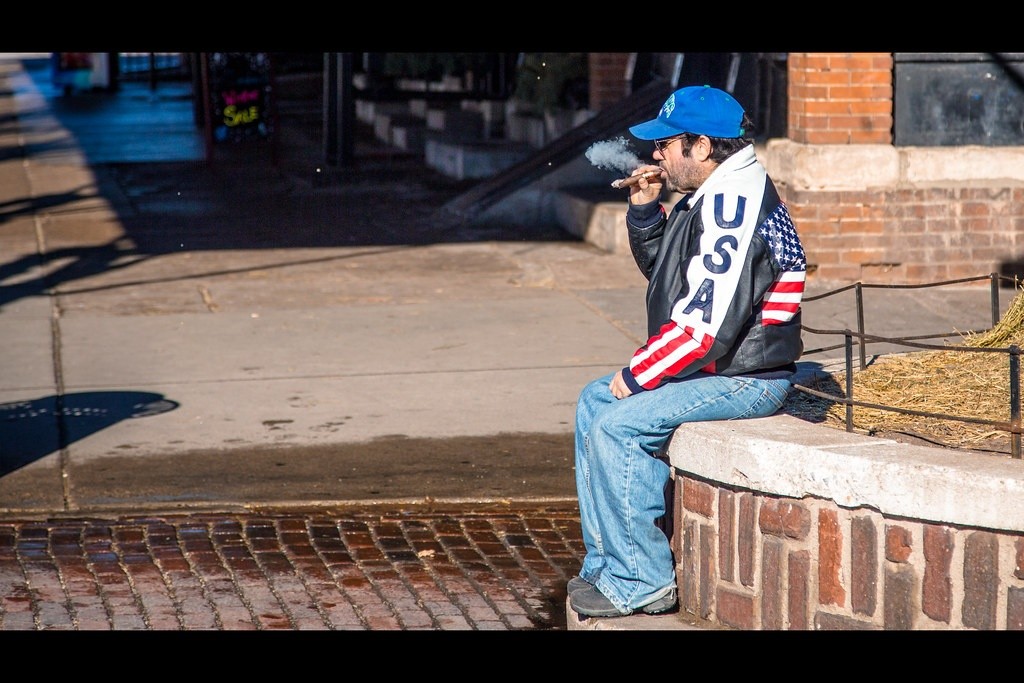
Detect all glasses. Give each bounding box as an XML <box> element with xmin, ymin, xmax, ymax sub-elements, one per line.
<box><xmin>652</xmin><ymin>137</ymin><xmax>697</xmax><ymax>160</ymax></box>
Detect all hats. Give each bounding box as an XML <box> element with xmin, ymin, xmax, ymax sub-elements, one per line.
<box><xmin>629</xmin><ymin>84</ymin><xmax>745</xmax><ymax>141</ymax></box>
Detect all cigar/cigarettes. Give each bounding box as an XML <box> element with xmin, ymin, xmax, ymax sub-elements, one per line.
<box><xmin>610</xmin><ymin>169</ymin><xmax>662</xmax><ymax>189</ymax></box>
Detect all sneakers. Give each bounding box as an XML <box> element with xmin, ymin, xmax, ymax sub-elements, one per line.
<box><xmin>567</xmin><ymin>576</ymin><xmax>594</xmax><ymax>596</ymax></box>
<box><xmin>569</xmin><ymin>584</ymin><xmax>677</xmax><ymax>617</ymax></box>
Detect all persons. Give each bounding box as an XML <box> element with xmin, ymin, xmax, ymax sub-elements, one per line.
<box><xmin>566</xmin><ymin>84</ymin><xmax>806</xmax><ymax>618</ymax></box>
